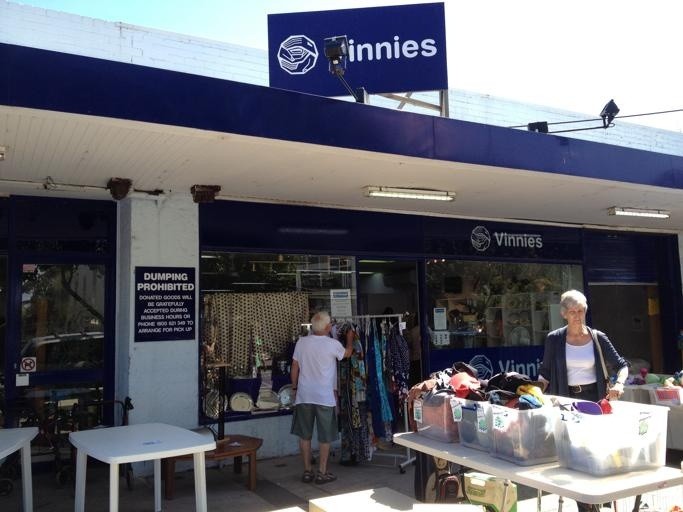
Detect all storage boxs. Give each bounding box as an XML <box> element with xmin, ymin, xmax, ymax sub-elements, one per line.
<box><xmin>414</xmin><ymin>374</ymin><xmax>683</xmax><ymax>475</ymax></box>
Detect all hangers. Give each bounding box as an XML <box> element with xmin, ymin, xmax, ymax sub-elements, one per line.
<box><xmin>330</xmin><ymin>311</ymin><xmax>393</xmax><ymax>341</ymax></box>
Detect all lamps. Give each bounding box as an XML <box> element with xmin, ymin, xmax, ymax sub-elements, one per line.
<box><xmin>528</xmin><ymin>99</ymin><xmax>620</xmax><ymax>133</ymax></box>
<box><xmin>323</xmin><ymin>34</ymin><xmax>369</xmax><ymax>105</ymax></box>
<box><xmin>360</xmin><ymin>184</ymin><xmax>457</xmax><ymax>202</ymax></box>
<box><xmin>607</xmin><ymin>206</ymin><xmax>673</xmax><ymax>219</ymax></box>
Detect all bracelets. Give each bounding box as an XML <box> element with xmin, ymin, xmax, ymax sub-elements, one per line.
<box><xmin>616</xmin><ymin>381</ymin><xmax>625</xmax><ymax>386</ymax></box>
<box><xmin>290</xmin><ymin>387</ymin><xmax>297</xmax><ymax>390</ymax></box>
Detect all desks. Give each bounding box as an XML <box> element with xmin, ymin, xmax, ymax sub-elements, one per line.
<box><xmin>161</xmin><ymin>435</ymin><xmax>263</xmax><ymax>500</ymax></box>
<box><xmin>69</xmin><ymin>423</ymin><xmax>217</xmax><ymax>512</ymax></box>
<box><xmin>0</xmin><ymin>427</ymin><xmax>39</xmax><ymax>512</ymax></box>
<box><xmin>393</xmin><ymin>431</ymin><xmax>683</xmax><ymax>512</ymax></box>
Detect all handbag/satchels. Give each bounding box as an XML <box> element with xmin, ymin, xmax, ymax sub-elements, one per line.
<box><xmin>606</xmin><ymin>383</ymin><xmax>618</xmax><ymax>401</ymax></box>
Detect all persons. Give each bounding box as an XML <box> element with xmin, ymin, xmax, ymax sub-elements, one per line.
<box><xmin>200</xmin><ymin>302</ymin><xmax>220</xmax><ymax>363</ymax></box>
<box><xmin>288</xmin><ymin>310</ymin><xmax>354</xmax><ymax>485</ymax></box>
<box><xmin>504</xmin><ymin>313</ymin><xmax>530</xmax><ymax>347</ymax></box>
<box><xmin>448</xmin><ymin>308</ymin><xmax>459</xmax><ymax>322</ymax></box>
<box><xmin>537</xmin><ymin>289</ymin><xmax>631</xmax><ymax>511</ymax></box>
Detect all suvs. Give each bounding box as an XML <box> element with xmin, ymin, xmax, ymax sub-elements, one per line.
<box><xmin>14</xmin><ymin>330</ymin><xmax>108</xmax><ymax>414</ymax></box>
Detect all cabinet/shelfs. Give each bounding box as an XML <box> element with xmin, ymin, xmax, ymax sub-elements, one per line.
<box><xmin>435</xmin><ymin>291</ymin><xmax>563</xmax><ymax>349</ymax></box>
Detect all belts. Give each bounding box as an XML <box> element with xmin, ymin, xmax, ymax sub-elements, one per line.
<box><xmin>571</xmin><ymin>385</ymin><xmax>582</xmax><ymax>394</ymax></box>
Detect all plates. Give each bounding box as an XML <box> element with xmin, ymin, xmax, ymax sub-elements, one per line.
<box><xmin>229</xmin><ymin>384</ymin><xmax>297</xmax><ymax>411</ymax></box>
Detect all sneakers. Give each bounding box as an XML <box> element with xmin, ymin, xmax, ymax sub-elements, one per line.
<box><xmin>316</xmin><ymin>470</ymin><xmax>337</xmax><ymax>484</ymax></box>
<box><xmin>303</xmin><ymin>471</ymin><xmax>314</xmax><ymax>483</ymax></box>
